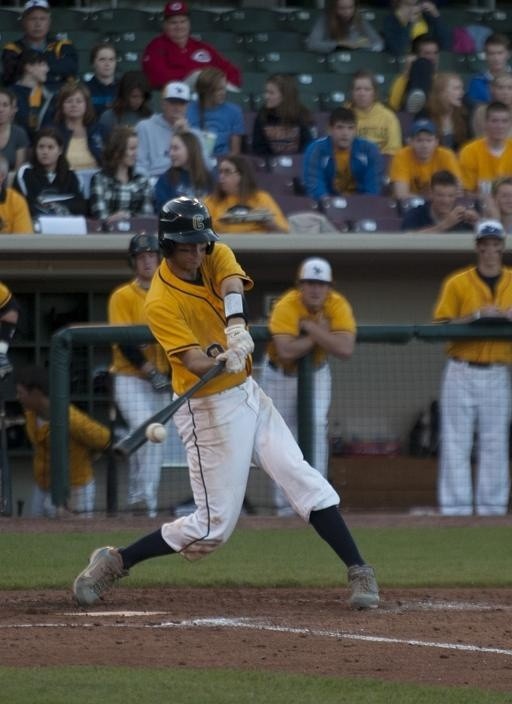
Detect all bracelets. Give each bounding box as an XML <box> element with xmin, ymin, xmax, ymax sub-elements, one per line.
<box><xmin>476</xmin><ymin>310</ymin><xmax>482</xmax><ymax>323</ymax></box>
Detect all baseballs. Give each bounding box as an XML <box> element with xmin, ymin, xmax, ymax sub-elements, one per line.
<box><xmin>145</xmin><ymin>423</ymin><xmax>169</xmax><ymax>443</ymax></box>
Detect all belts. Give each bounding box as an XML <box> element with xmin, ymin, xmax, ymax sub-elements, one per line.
<box><xmin>450</xmin><ymin>356</ymin><xmax>500</xmax><ymax>371</ymax></box>
<box><xmin>266</xmin><ymin>360</ymin><xmax>298</xmax><ymax>380</ymax></box>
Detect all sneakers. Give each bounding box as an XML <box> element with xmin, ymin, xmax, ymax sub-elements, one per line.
<box><xmin>72</xmin><ymin>545</ymin><xmax>130</xmax><ymax>607</ymax></box>
<box><xmin>347</xmin><ymin>562</ymin><xmax>380</xmax><ymax>610</ymax></box>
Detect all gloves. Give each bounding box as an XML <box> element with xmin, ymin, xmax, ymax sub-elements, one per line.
<box><xmin>213</xmin><ymin>348</ymin><xmax>247</xmax><ymax>375</ymax></box>
<box><xmin>0</xmin><ymin>351</ymin><xmax>13</xmax><ymax>382</ymax></box>
<box><xmin>146</xmin><ymin>369</ymin><xmax>170</xmax><ymax>393</ymax></box>
<box><xmin>225</xmin><ymin>324</ymin><xmax>255</xmax><ymax>357</ymax></box>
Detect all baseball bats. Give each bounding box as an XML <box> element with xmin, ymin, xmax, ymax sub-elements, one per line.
<box><xmin>112</xmin><ymin>360</ymin><xmax>229</xmax><ymax>461</ymax></box>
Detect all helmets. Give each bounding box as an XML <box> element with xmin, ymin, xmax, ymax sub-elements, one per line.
<box><xmin>158</xmin><ymin>196</ymin><xmax>220</xmax><ymax>258</ymax></box>
<box><xmin>128</xmin><ymin>232</ymin><xmax>161</xmax><ymax>270</ymax></box>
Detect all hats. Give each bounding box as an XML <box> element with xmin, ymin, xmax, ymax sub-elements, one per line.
<box><xmin>164</xmin><ymin>1</ymin><xmax>190</xmax><ymax>17</ymax></box>
<box><xmin>411</xmin><ymin>118</ymin><xmax>438</xmax><ymax>137</ymax></box>
<box><xmin>17</xmin><ymin>0</ymin><xmax>50</xmax><ymax>21</ymax></box>
<box><xmin>476</xmin><ymin>219</ymin><xmax>506</xmax><ymax>241</ymax></box>
<box><xmin>298</xmin><ymin>257</ymin><xmax>334</xmax><ymax>285</ymax></box>
<box><xmin>162</xmin><ymin>80</ymin><xmax>192</xmax><ymax>101</ymax></box>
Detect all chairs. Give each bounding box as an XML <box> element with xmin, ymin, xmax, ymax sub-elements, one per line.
<box><xmin>0</xmin><ymin>0</ymin><xmax>512</xmax><ymax>235</ymax></box>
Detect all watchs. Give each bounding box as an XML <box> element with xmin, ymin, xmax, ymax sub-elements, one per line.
<box><xmin>71</xmin><ymin>197</ymin><xmax>381</xmax><ymax>608</ymax></box>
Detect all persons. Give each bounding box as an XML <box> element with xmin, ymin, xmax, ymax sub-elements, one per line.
<box><xmin>71</xmin><ymin>197</ymin><xmax>381</xmax><ymax>608</ymax></box>
<box><xmin>431</xmin><ymin>217</ymin><xmax>510</xmax><ymax>522</ymax></box>
<box><xmin>14</xmin><ymin>370</ymin><xmax>115</xmax><ymax>520</ymax></box>
<box><xmin>104</xmin><ymin>231</ymin><xmax>164</xmax><ymax>519</ymax></box>
<box><xmin>261</xmin><ymin>257</ymin><xmax>357</xmax><ymax>515</ymax></box>
<box><xmin>0</xmin><ymin>279</ymin><xmax>21</xmax><ymax>378</ymax></box>
<box><xmin>0</xmin><ymin>2</ymin><xmax>510</xmax><ymax>235</ymax></box>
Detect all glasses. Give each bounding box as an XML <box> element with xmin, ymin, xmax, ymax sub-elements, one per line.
<box><xmin>216</xmin><ymin>167</ymin><xmax>239</xmax><ymax>177</ymax></box>
